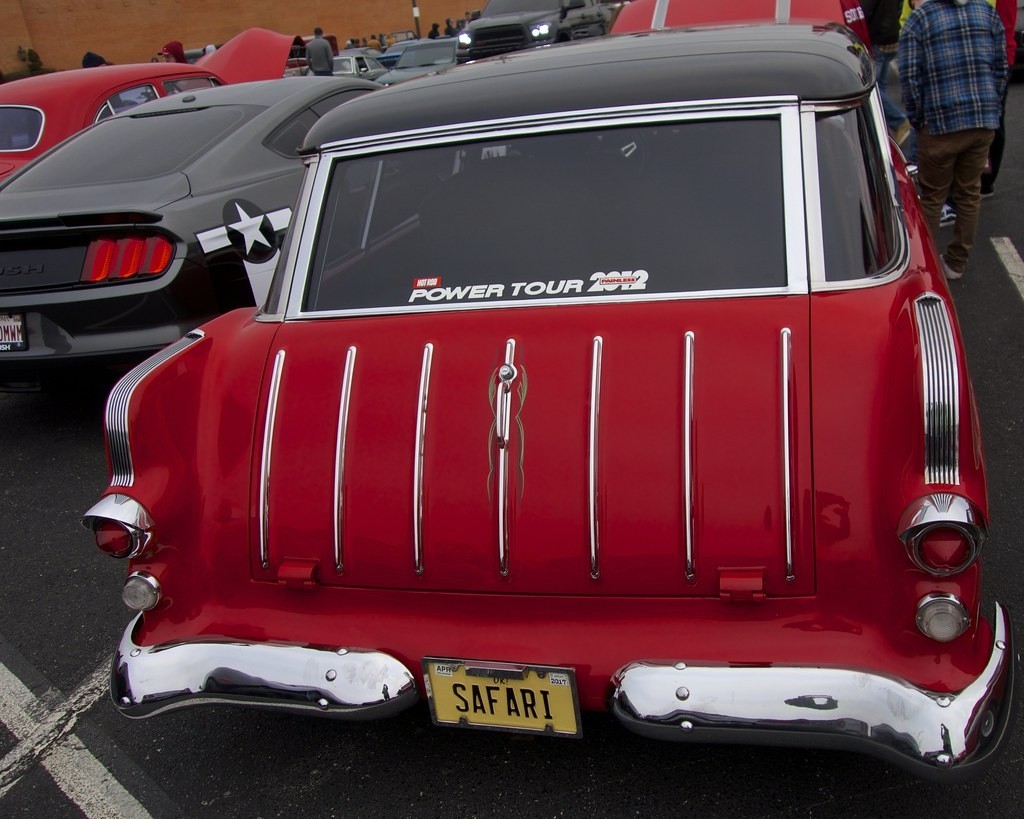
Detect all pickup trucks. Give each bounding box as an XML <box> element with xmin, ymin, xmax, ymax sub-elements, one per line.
<box><xmin>457</xmin><ymin>0</ymin><xmax>621</xmax><ymax>65</ymax></box>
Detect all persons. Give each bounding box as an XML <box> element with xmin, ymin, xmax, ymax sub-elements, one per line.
<box><xmin>344</xmin><ymin>38</ymin><xmax>360</xmax><ymax>48</ymax></box>
<box><xmin>362</xmin><ymin>38</ymin><xmax>367</xmax><ymax>47</ymax></box>
<box><xmin>306</xmin><ymin>27</ymin><xmax>334</xmax><ymax>76</ymax></box>
<box><xmin>366</xmin><ymin>32</ymin><xmax>397</xmax><ymax>51</ymax></box>
<box><xmin>17</xmin><ymin>45</ymin><xmax>26</xmax><ymax>63</ymax></box>
<box><xmin>151</xmin><ymin>56</ymin><xmax>159</xmax><ymax>62</ymax></box>
<box><xmin>839</xmin><ymin>0</ymin><xmax>1018</xmax><ymax>282</ymax></box>
<box><xmin>157</xmin><ymin>40</ymin><xmax>190</xmax><ymax>64</ymax></box>
<box><xmin>444</xmin><ymin>17</ymin><xmax>464</xmax><ymax>38</ymax></box>
<box><xmin>462</xmin><ymin>11</ymin><xmax>472</xmax><ymax>30</ymax></box>
<box><xmin>202</xmin><ymin>44</ymin><xmax>220</xmax><ymax>54</ymax></box>
<box><xmin>428</xmin><ymin>23</ymin><xmax>440</xmax><ymax>40</ymax></box>
<box><xmin>82</xmin><ymin>52</ymin><xmax>113</xmax><ymax>68</ymax></box>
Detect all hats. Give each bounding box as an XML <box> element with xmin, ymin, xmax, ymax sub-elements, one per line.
<box><xmin>157</xmin><ymin>47</ymin><xmax>170</xmax><ymax>55</ymax></box>
<box><xmin>82</xmin><ymin>51</ymin><xmax>105</xmax><ymax>68</ymax></box>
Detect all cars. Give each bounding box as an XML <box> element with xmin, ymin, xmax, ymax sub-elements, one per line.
<box><xmin>1</xmin><ymin>27</ymin><xmax>310</xmax><ymax>191</ymax></box>
<box><xmin>82</xmin><ymin>0</ymin><xmax>1015</xmax><ymax>781</ymax></box>
<box><xmin>183</xmin><ymin>34</ymin><xmax>459</xmax><ymax>87</ymax></box>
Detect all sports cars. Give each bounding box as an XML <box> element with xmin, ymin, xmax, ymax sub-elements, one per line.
<box><xmin>0</xmin><ymin>75</ymin><xmax>475</xmax><ymax>402</ymax></box>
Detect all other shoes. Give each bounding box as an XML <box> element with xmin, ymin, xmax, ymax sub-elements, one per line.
<box><xmin>896</xmin><ymin>120</ymin><xmax>911</xmax><ymax>145</ymax></box>
<box><xmin>939</xmin><ymin>204</ymin><xmax>957</xmax><ymax>227</ymax></box>
<box><xmin>939</xmin><ymin>254</ymin><xmax>962</xmax><ymax>280</ymax></box>
<box><xmin>980</xmin><ymin>186</ymin><xmax>993</xmax><ymax>198</ymax></box>
